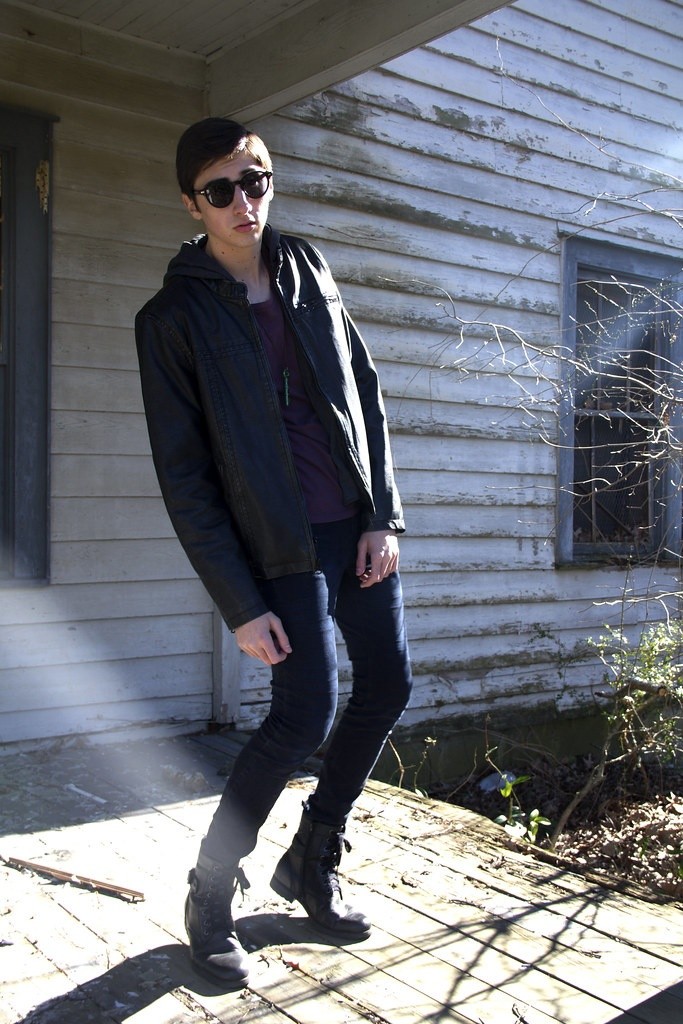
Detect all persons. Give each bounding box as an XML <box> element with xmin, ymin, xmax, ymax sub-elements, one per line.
<box><xmin>132</xmin><ymin>116</ymin><xmax>415</xmax><ymax>992</ymax></box>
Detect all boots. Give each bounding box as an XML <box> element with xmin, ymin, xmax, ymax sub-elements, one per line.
<box><xmin>270</xmin><ymin>800</ymin><xmax>372</xmax><ymax>941</ymax></box>
<box><xmin>184</xmin><ymin>836</ymin><xmax>250</xmax><ymax>989</ymax></box>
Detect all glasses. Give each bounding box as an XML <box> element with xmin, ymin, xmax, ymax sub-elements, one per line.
<box><xmin>194</xmin><ymin>170</ymin><xmax>273</xmax><ymax>208</ymax></box>
